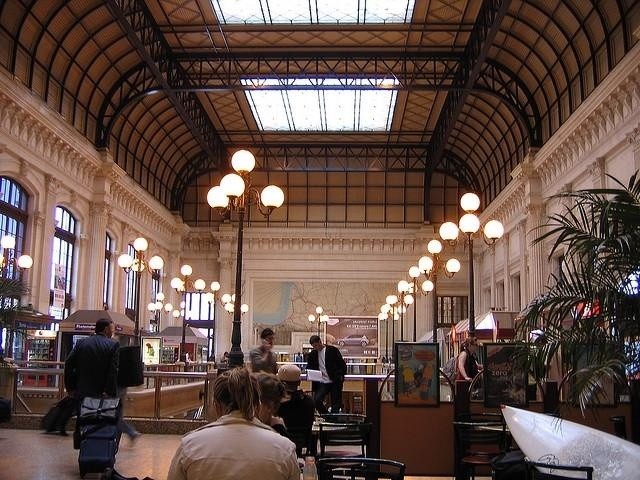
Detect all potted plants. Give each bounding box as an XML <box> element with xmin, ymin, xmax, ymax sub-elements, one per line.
<box><xmin>503</xmin><ymin>165</ymin><xmax>640</xmax><ymax>447</ymax></box>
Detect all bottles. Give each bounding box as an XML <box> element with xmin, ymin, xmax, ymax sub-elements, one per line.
<box><xmin>420</xmin><ymin>362</ymin><xmax>432</xmax><ymax>397</ymax></box>
<box><xmin>401</xmin><ymin>362</ymin><xmax>417</xmax><ymax>398</ymax></box>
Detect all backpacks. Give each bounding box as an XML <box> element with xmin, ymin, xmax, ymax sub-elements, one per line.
<box><xmin>441</xmin><ymin>355</ymin><xmax>469</xmax><ymax>380</ymax></box>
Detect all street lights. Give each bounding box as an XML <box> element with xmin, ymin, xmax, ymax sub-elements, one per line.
<box><xmin>117</xmin><ymin>236</ymin><xmax>165</xmax><ymax>344</ymax></box>
<box><xmin>376</xmin><ymin>264</ymin><xmax>432</xmax><ymax>363</ymax></box>
<box><xmin>0</xmin><ymin>233</ymin><xmax>34</xmax><ymax>275</ymax></box>
<box><xmin>308</xmin><ymin>306</ymin><xmax>329</xmax><ymax>337</ymax></box>
<box><xmin>437</xmin><ymin>188</ymin><xmax>507</xmax><ymax>337</ymax></box>
<box><xmin>147</xmin><ymin>290</ymin><xmax>173</xmax><ymax>335</ymax></box>
<box><xmin>204</xmin><ymin>147</ymin><xmax>287</xmax><ymax>367</ymax></box>
<box><xmin>418</xmin><ymin>239</ymin><xmax>461</xmax><ymax>343</ymax></box>
<box><xmin>164</xmin><ymin>262</ymin><xmax>250</xmax><ymax>362</ymax></box>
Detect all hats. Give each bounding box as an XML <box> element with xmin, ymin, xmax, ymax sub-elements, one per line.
<box><xmin>276</xmin><ymin>365</ymin><xmax>301</xmax><ymax>382</ymax></box>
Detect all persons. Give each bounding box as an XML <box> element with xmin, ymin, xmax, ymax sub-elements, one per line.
<box><xmin>62</xmin><ymin>317</ymin><xmax>122</xmax><ymax>422</ymax></box>
<box><xmin>295</xmin><ymin>350</ymin><xmax>304</xmax><ymax>370</ymax></box>
<box><xmin>186</xmin><ymin>358</ymin><xmax>195</xmax><ymax>369</ymax></box>
<box><xmin>166</xmin><ymin>364</ymin><xmax>303</xmax><ymax>479</ymax></box>
<box><xmin>220</xmin><ymin>352</ymin><xmax>228</xmax><ymax>362</ymax></box>
<box><xmin>306</xmin><ymin>335</ymin><xmax>348</xmax><ymax>415</ymax></box>
<box><xmin>253</xmin><ymin>370</ymin><xmax>291</xmax><ymax>441</ymax></box>
<box><xmin>118</xmin><ymin>417</ymin><xmax>143</xmax><ymax>452</ymax></box>
<box><xmin>248</xmin><ymin>327</ymin><xmax>277</xmax><ymax>418</ymax></box>
<box><xmin>452</xmin><ymin>336</ymin><xmax>484</xmax><ymax>395</ymax></box>
<box><xmin>185</xmin><ymin>351</ymin><xmax>193</xmax><ymax>361</ymax></box>
<box><xmin>276</xmin><ymin>364</ymin><xmax>316</xmax><ymax>463</ymax></box>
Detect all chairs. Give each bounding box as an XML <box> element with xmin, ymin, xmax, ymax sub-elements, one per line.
<box><xmin>308</xmin><ymin>412</ymin><xmax>598</xmax><ymax>479</ymax></box>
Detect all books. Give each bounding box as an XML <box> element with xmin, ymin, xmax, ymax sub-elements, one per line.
<box><xmin>304</xmin><ymin>368</ymin><xmax>323</xmax><ymax>382</ymax></box>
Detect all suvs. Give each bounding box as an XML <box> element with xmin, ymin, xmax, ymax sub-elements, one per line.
<box><xmin>335</xmin><ymin>332</ymin><xmax>371</xmax><ymax>349</ymax></box>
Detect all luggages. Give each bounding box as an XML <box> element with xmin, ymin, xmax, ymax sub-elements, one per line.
<box><xmin>41</xmin><ymin>395</ymin><xmax>119</xmax><ymax>478</ymax></box>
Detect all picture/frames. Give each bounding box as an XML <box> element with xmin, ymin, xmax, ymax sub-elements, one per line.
<box><xmin>569</xmin><ymin>341</ymin><xmax>620</xmax><ymax>409</ymax></box>
<box><xmin>324</xmin><ymin>315</ymin><xmax>382</xmax><ymax>358</ymax></box>
<box><xmin>141</xmin><ymin>336</ymin><xmax>162</xmax><ymax>365</ymax></box>
<box><xmin>395</xmin><ymin>342</ymin><xmax>440</xmax><ymax>408</ymax></box>
<box><xmin>484</xmin><ymin>342</ymin><xmax>529</xmax><ymax>409</ymax></box>
